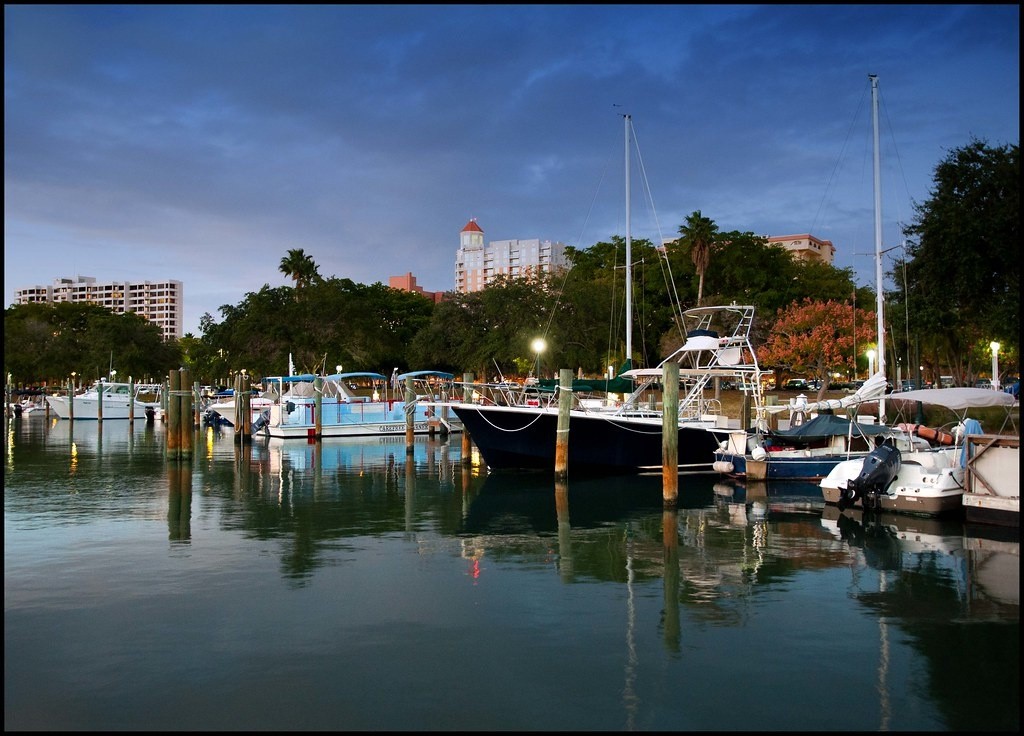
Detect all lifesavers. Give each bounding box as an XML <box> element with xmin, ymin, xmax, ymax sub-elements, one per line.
<box><xmin>898</xmin><ymin>423</ymin><xmax>952</xmax><ymax>444</ymax></box>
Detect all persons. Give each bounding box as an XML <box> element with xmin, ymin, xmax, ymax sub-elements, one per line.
<box><xmin>144</xmin><ymin>406</ymin><xmax>156</xmax><ymax>422</ymax></box>
<box><xmin>250</xmin><ymin>381</ymin><xmax>289</xmax><ymax>394</ymax></box>
<box><xmin>375</xmin><ymin>363</ymin><xmax>615</xmax><ymax>391</ymax></box>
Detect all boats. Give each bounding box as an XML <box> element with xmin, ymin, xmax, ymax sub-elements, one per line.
<box><xmin>959</xmin><ymin>434</ymin><xmax>1020</xmax><ymax>528</ymax></box>
<box><xmin>43</xmin><ymin>352</ymin><xmax>168</xmax><ymax>420</ymax></box>
<box><xmin>210</xmin><ymin>354</ymin><xmax>465</xmax><ymax>439</ymax></box>
<box><xmin>821</xmin><ymin>387</ymin><xmax>1014</xmax><ymax>513</ymax></box>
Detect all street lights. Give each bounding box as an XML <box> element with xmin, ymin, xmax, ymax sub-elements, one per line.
<box><xmin>864</xmin><ymin>349</ymin><xmax>875</xmax><ymax>380</ymax></box>
<box><xmin>533</xmin><ymin>339</ymin><xmax>547</xmax><ymax>408</ymax></box>
<box><xmin>989</xmin><ymin>342</ymin><xmax>1002</xmax><ymax>391</ymax></box>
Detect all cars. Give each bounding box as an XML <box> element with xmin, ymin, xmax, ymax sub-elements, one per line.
<box><xmin>679</xmin><ymin>375</ymin><xmax>1019</xmax><ymax>398</ymax></box>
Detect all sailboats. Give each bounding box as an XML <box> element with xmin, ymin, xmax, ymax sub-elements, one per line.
<box><xmin>709</xmin><ymin>73</ymin><xmax>937</xmax><ymax>482</ymax></box>
<box><xmin>416</xmin><ymin>103</ymin><xmax>770</xmax><ymax>479</ymax></box>
